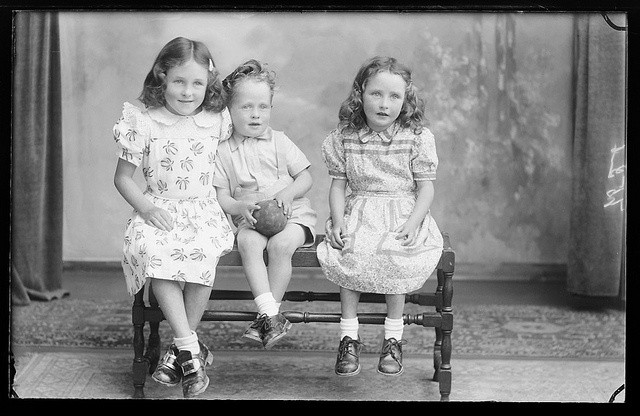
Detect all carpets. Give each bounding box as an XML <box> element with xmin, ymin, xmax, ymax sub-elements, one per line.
<box><xmin>8</xmin><ymin>294</ymin><xmax>626</xmax><ymax>359</ymax></box>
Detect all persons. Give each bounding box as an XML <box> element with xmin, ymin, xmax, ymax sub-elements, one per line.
<box><xmin>213</xmin><ymin>59</ymin><xmax>315</xmax><ymax>350</ymax></box>
<box><xmin>114</xmin><ymin>37</ymin><xmax>232</xmax><ymax>398</ymax></box>
<box><xmin>316</xmin><ymin>55</ymin><xmax>445</xmax><ymax>378</ymax></box>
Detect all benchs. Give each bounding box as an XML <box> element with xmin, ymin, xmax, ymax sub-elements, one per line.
<box><xmin>129</xmin><ymin>222</ymin><xmax>457</xmax><ymax>402</ymax></box>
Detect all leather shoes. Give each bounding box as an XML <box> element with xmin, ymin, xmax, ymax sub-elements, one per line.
<box><xmin>334</xmin><ymin>335</ymin><xmax>362</xmax><ymax>377</ymax></box>
<box><xmin>259</xmin><ymin>313</ymin><xmax>291</xmax><ymax>348</ymax></box>
<box><xmin>241</xmin><ymin>313</ymin><xmax>263</xmax><ymax>345</ymax></box>
<box><xmin>152</xmin><ymin>343</ymin><xmax>183</xmax><ymax>386</ymax></box>
<box><xmin>378</xmin><ymin>339</ymin><xmax>408</xmax><ymax>375</ymax></box>
<box><xmin>176</xmin><ymin>342</ymin><xmax>214</xmax><ymax>399</ymax></box>
